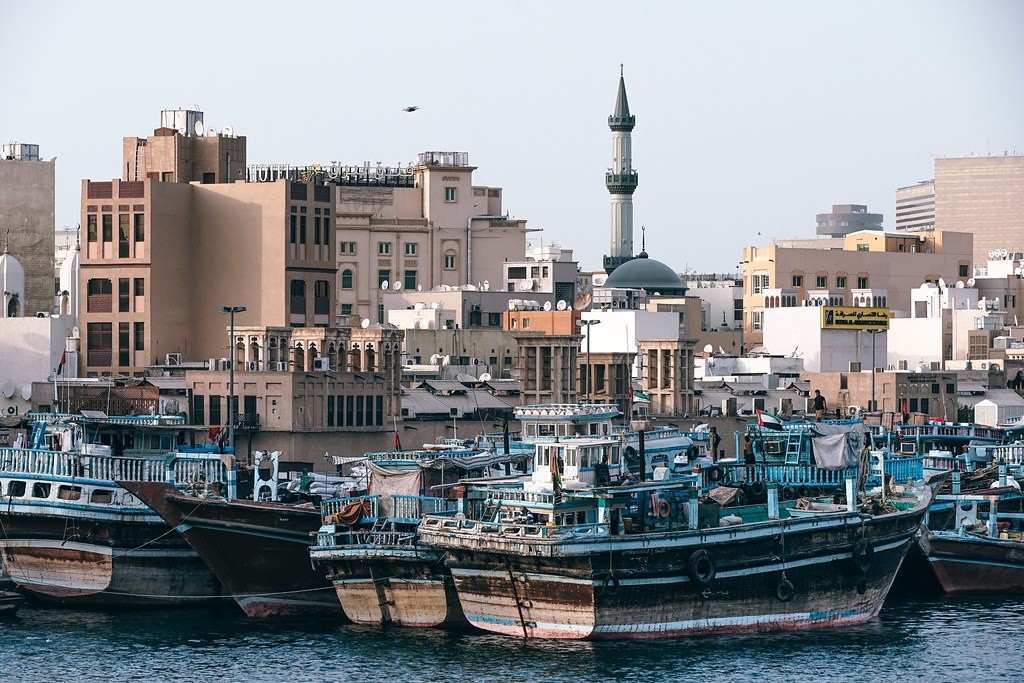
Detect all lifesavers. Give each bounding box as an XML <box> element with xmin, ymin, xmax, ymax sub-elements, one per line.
<box><xmin>601</xmin><ymin>576</ymin><xmax>621</xmax><ymax>597</ymax></box>
<box><xmin>858</xmin><ymin>580</ymin><xmax>867</xmax><ymax>595</ymax></box>
<box><xmin>688</xmin><ymin>549</ymin><xmax>719</xmax><ymax>588</ymax></box>
<box><xmin>709</xmin><ymin>467</ymin><xmax>722</xmax><ymax>481</ymax></box>
<box><xmin>687</xmin><ymin>444</ymin><xmax>699</xmax><ymax>461</ymax></box>
<box><xmin>655</xmin><ymin>500</ymin><xmax>671</xmax><ymax>519</ymax></box>
<box><xmin>853</xmin><ymin>538</ymin><xmax>874</xmax><ymax>572</ymax></box>
<box><xmin>623</xmin><ymin>444</ymin><xmax>638</xmax><ymax>461</ymax></box>
<box><xmin>775</xmin><ymin>580</ymin><xmax>793</xmax><ymax>602</ymax></box>
<box><xmin>739</xmin><ymin>479</ymin><xmax>824</xmax><ymax>501</ymax></box>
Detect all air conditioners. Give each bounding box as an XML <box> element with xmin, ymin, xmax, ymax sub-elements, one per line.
<box><xmin>449</xmin><ymin>407</ymin><xmax>463</xmax><ymax>417</ymax></box>
<box><xmin>804</xmin><ymin>397</ymin><xmax>816</xmax><ymax>414</ymax></box>
<box><xmin>848</xmin><ymin>361</ymin><xmax>861</xmax><ymax>373</ymax></box>
<box><xmin>5</xmin><ymin>406</ymin><xmax>19</xmax><ymax>417</ymax></box>
<box><xmin>818</xmin><ymin>299</ymin><xmax>826</xmax><ymax>306</ymax></box>
<box><xmin>36</xmin><ymin>311</ymin><xmax>51</xmax><ymax>319</ymax></box>
<box><xmin>900</xmin><ymin>443</ymin><xmax>917</xmax><ymax>456</ymax></box>
<box><xmin>763</xmin><ymin>440</ymin><xmax>785</xmax><ymax>454</ymax></box>
<box><xmin>875</xmin><ymin>367</ymin><xmax>885</xmax><ymax>373</ymax></box>
<box><xmin>245</xmin><ymin>361</ymin><xmax>257</xmax><ymax>372</ymax></box>
<box><xmin>400</xmin><ymin>407</ymin><xmax>414</xmax><ymax>419</ymax></box>
<box><xmin>277</xmin><ymin>362</ymin><xmax>287</xmax><ymax>371</ymax></box>
<box><xmin>314</xmin><ymin>357</ymin><xmax>329</xmax><ymax>372</ymax></box>
<box><xmin>471</xmin><ymin>357</ymin><xmax>482</xmax><ymax>365</ymax></box>
<box><xmin>898</xmin><ymin>360</ymin><xmax>908</xmax><ymax>370</ymax></box>
<box><xmin>165</xmin><ymin>353</ymin><xmax>182</xmax><ymax>365</ymax></box>
<box><xmin>807</xmin><ymin>299</ymin><xmax>815</xmax><ymax>307</ymax></box>
<box><xmin>219</xmin><ymin>358</ymin><xmax>231</xmax><ymax>371</ymax></box>
<box><xmin>405</xmin><ymin>359</ymin><xmax>416</xmax><ymax>365</ymax></box>
<box><xmin>847</xmin><ymin>405</ymin><xmax>859</xmax><ymax>417</ymax></box>
<box><xmin>780</xmin><ymin>397</ymin><xmax>791</xmax><ymax>414</ymax></box>
<box><xmin>930</xmin><ymin>361</ymin><xmax>940</xmax><ymax>371</ymax></box>
<box><xmin>752</xmin><ymin>398</ymin><xmax>766</xmax><ymax>415</ymax></box>
<box><xmin>981</xmin><ymin>362</ymin><xmax>990</xmax><ymax>370</ymax></box>
<box><xmin>472</xmin><ymin>304</ymin><xmax>481</xmax><ymax>311</ymax></box>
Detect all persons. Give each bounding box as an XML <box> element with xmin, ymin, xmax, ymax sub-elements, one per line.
<box><xmin>512</xmin><ymin>506</ymin><xmax>534</xmax><ymax>525</ymax></box>
<box><xmin>899</xmin><ymin>433</ymin><xmax>905</xmax><ymax>442</ymax></box>
<box><xmin>744</xmin><ymin>425</ymin><xmax>757</xmax><ymax>483</ymax></box>
<box><xmin>811</xmin><ymin>390</ymin><xmax>827</xmax><ymax>423</ymax></box>
<box><xmin>1012</xmin><ymin>367</ymin><xmax>1024</xmax><ymax>390</ymax></box>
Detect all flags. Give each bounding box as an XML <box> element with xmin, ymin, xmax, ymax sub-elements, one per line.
<box><xmin>632</xmin><ymin>390</ymin><xmax>651</xmax><ymax>405</ymax></box>
<box><xmin>56</xmin><ymin>363</ymin><xmax>62</xmax><ymax>375</ymax></box>
<box><xmin>756</xmin><ymin>409</ymin><xmax>783</xmax><ymax>431</ymax></box>
<box><xmin>60</xmin><ymin>351</ymin><xmax>67</xmax><ymax>364</ymax></box>
<box><xmin>553</xmin><ymin>454</ymin><xmax>563</xmax><ymax>487</ymax></box>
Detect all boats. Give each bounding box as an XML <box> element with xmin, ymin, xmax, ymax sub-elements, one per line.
<box><xmin>0</xmin><ymin>403</ymin><xmax>1024</xmax><ymax>640</ymax></box>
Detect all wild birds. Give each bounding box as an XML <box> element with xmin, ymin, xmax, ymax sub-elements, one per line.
<box><xmin>402</xmin><ymin>105</ymin><xmax>422</xmax><ymax>112</ymax></box>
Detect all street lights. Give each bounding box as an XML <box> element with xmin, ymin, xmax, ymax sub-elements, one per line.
<box><xmin>860</xmin><ymin>327</ymin><xmax>886</xmax><ymax>411</ymax></box>
<box><xmin>577</xmin><ymin>319</ymin><xmax>601</xmax><ymax>403</ymax></box>
<box><xmin>215</xmin><ymin>304</ymin><xmax>247</xmax><ymax>455</ymax></box>
